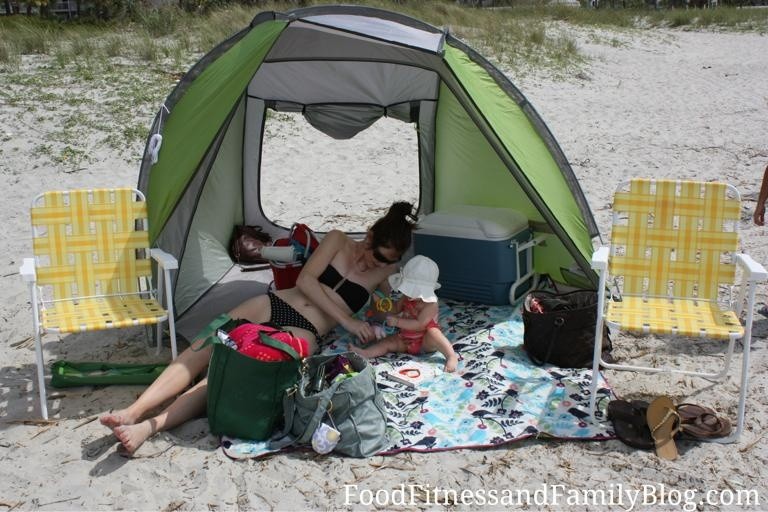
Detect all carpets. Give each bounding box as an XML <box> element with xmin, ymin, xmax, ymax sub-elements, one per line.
<box><xmin>218</xmin><ymin>297</ymin><xmax>627</xmax><ymax>464</ymax></box>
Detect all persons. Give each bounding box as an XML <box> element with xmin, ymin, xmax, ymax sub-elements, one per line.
<box><xmin>348</xmin><ymin>254</ymin><xmax>458</xmax><ymax>374</ymax></box>
<box><xmin>753</xmin><ymin>166</ymin><xmax>767</xmax><ymax>318</ymax></box>
<box><xmin>366</xmin><ymin>290</ymin><xmax>396</xmax><ymax>335</ymax></box>
<box><xmin>97</xmin><ymin>197</ymin><xmax>424</xmax><ymax>455</ymax></box>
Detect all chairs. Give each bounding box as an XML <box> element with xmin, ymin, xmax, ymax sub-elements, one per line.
<box><xmin>591</xmin><ymin>177</ymin><xmax>767</xmax><ymax>447</ymax></box>
<box><xmin>19</xmin><ymin>185</ymin><xmax>181</xmax><ymax>422</ymax></box>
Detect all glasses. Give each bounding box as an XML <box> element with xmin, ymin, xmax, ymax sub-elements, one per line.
<box><xmin>371</xmin><ymin>242</ymin><xmax>402</xmax><ymax>264</ymax></box>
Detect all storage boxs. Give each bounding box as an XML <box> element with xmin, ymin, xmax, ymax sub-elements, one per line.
<box><xmin>412</xmin><ymin>205</ymin><xmax>532</xmax><ymax>306</ymax></box>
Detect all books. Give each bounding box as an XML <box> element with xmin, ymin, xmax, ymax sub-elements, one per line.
<box><xmin>388</xmin><ymin>360</ymin><xmax>435</xmax><ymax>386</ymax></box>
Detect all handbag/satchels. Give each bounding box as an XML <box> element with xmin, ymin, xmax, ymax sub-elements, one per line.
<box><xmin>522</xmin><ymin>288</ymin><xmax>620</xmax><ymax>370</ymax></box>
<box><xmin>227</xmin><ymin>224</ymin><xmax>273</xmax><ymax>265</ymax></box>
<box><xmin>206</xmin><ymin>318</ymin><xmax>389</xmax><ymax>458</ymax></box>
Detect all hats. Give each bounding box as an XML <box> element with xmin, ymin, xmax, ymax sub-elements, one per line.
<box><xmin>388</xmin><ymin>254</ymin><xmax>442</xmax><ymax>303</ymax></box>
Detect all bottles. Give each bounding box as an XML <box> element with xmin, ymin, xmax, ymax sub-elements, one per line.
<box><xmin>530</xmin><ymin>299</ymin><xmax>544</xmax><ymax>314</ymax></box>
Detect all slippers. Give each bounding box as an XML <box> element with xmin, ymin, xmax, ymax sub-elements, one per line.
<box><xmin>608</xmin><ymin>396</ymin><xmax>731</xmax><ymax>461</ymax></box>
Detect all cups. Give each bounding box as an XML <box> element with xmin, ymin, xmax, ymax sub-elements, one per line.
<box><xmin>261</xmin><ymin>246</ymin><xmax>297</xmax><ymax>264</ymax></box>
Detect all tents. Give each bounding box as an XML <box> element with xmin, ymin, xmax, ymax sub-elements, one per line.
<box><xmin>132</xmin><ymin>3</ymin><xmax>623</xmax><ymax>348</ymax></box>
